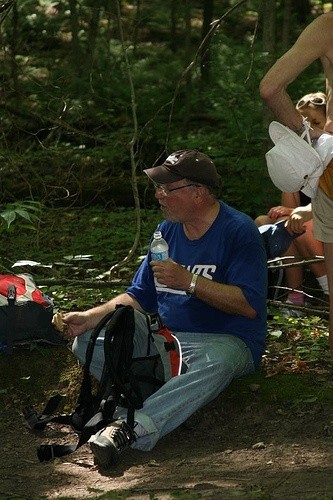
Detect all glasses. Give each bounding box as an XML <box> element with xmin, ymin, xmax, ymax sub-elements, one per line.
<box><xmin>296</xmin><ymin>97</ymin><xmax>326</xmax><ymax>110</ymax></box>
<box><xmin>156</xmin><ymin>184</ymin><xmax>200</xmax><ymax>196</ymax></box>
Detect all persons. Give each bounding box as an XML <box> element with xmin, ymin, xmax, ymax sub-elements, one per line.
<box><xmin>251</xmin><ymin>7</ymin><xmax>333</xmax><ymax>352</ymax></box>
<box><xmin>61</xmin><ymin>150</ymin><xmax>268</xmax><ymax>464</ymax></box>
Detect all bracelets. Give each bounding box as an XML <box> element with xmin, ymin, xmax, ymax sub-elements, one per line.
<box><xmin>294</xmin><ymin>123</ymin><xmax>305</xmax><ymax>133</ymax></box>
<box><xmin>188</xmin><ymin>274</ymin><xmax>198</xmax><ymax>293</ymax></box>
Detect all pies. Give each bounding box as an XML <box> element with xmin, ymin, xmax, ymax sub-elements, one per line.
<box><xmin>52</xmin><ymin>311</ymin><xmax>65</xmax><ymax>331</ymax></box>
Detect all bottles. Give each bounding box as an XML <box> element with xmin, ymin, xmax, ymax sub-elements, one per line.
<box><xmin>150</xmin><ymin>232</ymin><xmax>169</xmax><ymax>288</ymax></box>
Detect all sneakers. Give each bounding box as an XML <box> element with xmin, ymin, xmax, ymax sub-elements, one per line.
<box><xmin>87</xmin><ymin>420</ymin><xmax>137</xmax><ymax>466</ymax></box>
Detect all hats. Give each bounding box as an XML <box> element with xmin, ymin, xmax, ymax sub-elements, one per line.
<box><xmin>143</xmin><ymin>149</ymin><xmax>219</xmax><ymax>185</ymax></box>
<box><xmin>264</xmin><ymin>120</ymin><xmax>323</xmax><ymax>193</ymax></box>
<box><xmin>257</xmin><ymin>219</ymin><xmax>305</xmax><ymax>258</ymax></box>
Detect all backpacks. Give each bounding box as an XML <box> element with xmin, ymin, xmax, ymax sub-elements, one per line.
<box><xmin>68</xmin><ymin>304</ymin><xmax>182</xmax><ymax>435</ymax></box>
<box><xmin>0</xmin><ymin>272</ymin><xmax>52</xmax><ymax>341</ymax></box>
